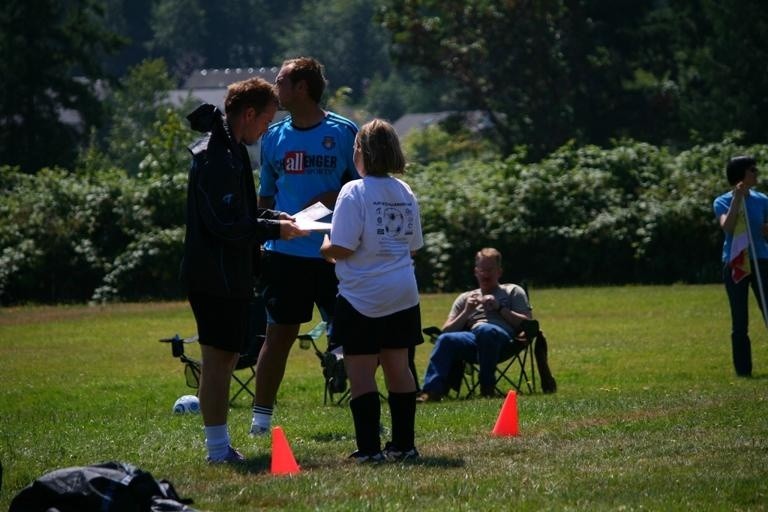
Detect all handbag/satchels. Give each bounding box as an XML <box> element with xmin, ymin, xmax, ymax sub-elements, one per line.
<box><xmin>8</xmin><ymin>460</ymin><xmax>201</xmax><ymax>512</ymax></box>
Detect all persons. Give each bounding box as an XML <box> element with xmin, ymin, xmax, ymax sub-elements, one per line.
<box><xmin>250</xmin><ymin>55</ymin><xmax>364</xmax><ymax>436</ymax></box>
<box><xmin>181</xmin><ymin>74</ymin><xmax>281</xmax><ymax>464</ymax></box>
<box><xmin>712</xmin><ymin>155</ymin><xmax>767</xmax><ymax>375</ymax></box>
<box><xmin>414</xmin><ymin>246</ymin><xmax>535</xmax><ymax>401</ymax></box>
<box><xmin>317</xmin><ymin>116</ymin><xmax>426</xmax><ymax>461</ymax></box>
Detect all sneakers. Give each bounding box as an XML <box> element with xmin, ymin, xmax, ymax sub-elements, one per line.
<box><xmin>350</xmin><ymin>442</ymin><xmax>419</xmax><ymax>462</ymax></box>
<box><xmin>249</xmin><ymin>426</ymin><xmax>269</xmax><ymax>437</ymax></box>
<box><xmin>416</xmin><ymin>390</ymin><xmax>441</xmax><ymax>403</ymax></box>
<box><xmin>207</xmin><ymin>447</ymin><xmax>245</xmax><ymax>462</ymax></box>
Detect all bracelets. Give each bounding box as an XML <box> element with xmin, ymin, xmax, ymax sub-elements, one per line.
<box><xmin>494</xmin><ymin>301</ymin><xmax>505</xmax><ymax>314</ymax></box>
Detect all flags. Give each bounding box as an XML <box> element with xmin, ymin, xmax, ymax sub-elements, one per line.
<box><xmin>728</xmin><ymin>188</ymin><xmax>753</xmax><ymax>286</ymax></box>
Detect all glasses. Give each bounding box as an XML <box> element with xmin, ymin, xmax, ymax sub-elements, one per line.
<box><xmin>751</xmin><ymin>166</ymin><xmax>758</xmax><ymax>173</ymax></box>
<box><xmin>476</xmin><ymin>269</ymin><xmax>499</xmax><ymax>274</ymax></box>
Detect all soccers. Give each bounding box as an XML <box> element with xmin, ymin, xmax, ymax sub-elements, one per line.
<box><xmin>174</xmin><ymin>395</ymin><xmax>202</xmax><ymax>413</ymax></box>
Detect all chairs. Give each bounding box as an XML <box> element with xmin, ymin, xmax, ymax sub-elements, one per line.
<box><xmin>297</xmin><ymin>321</ymin><xmax>420</xmax><ymax>405</ymax></box>
<box><xmin>423</xmin><ymin>282</ymin><xmax>537</xmax><ymax>402</ymax></box>
<box><xmin>158</xmin><ymin>289</ymin><xmax>278</xmax><ymax>409</ymax></box>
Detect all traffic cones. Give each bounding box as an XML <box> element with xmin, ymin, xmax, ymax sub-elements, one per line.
<box><xmin>271</xmin><ymin>427</ymin><xmax>299</xmax><ymax>474</ymax></box>
<box><xmin>491</xmin><ymin>390</ymin><xmax>517</xmax><ymax>436</ymax></box>
